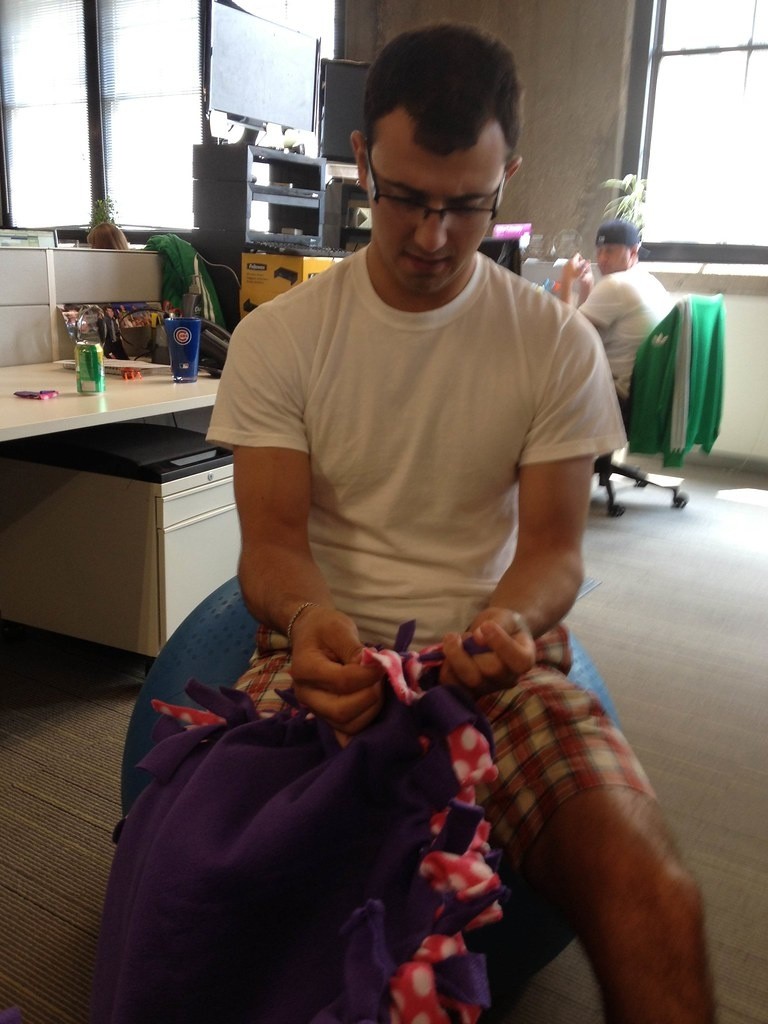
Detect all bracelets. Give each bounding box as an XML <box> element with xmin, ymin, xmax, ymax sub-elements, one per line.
<box><xmin>288</xmin><ymin>602</ymin><xmax>324</xmax><ymax>642</ymax></box>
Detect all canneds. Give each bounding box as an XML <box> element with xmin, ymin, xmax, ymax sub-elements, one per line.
<box><xmin>74</xmin><ymin>340</ymin><xmax>106</xmax><ymax>396</ymax></box>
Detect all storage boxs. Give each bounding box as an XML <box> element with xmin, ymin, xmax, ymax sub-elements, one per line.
<box><xmin>239</xmin><ymin>251</ymin><xmax>345</xmax><ymax>323</ymax></box>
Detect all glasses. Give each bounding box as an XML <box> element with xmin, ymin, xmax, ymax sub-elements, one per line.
<box><xmin>364</xmin><ymin>139</ymin><xmax>507</xmax><ymax>230</ymax></box>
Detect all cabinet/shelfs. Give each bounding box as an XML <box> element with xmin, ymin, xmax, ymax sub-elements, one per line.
<box><xmin>0</xmin><ymin>432</ymin><xmax>245</xmax><ymax>681</ymax></box>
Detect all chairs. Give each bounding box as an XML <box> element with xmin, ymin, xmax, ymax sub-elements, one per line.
<box><xmin>595</xmin><ymin>292</ymin><xmax>726</xmax><ymax>517</ymax></box>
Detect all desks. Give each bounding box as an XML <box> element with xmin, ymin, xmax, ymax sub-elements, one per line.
<box><xmin>0</xmin><ymin>356</ymin><xmax>235</xmax><ymax>443</ymax></box>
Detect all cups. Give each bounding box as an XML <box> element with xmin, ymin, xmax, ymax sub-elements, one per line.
<box><xmin>165</xmin><ymin>317</ymin><xmax>201</xmax><ymax>383</ymax></box>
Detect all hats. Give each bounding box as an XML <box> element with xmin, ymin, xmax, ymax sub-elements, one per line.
<box><xmin>596</xmin><ymin>219</ymin><xmax>641</xmax><ymax>247</ymax></box>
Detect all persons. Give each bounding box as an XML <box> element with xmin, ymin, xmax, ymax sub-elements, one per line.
<box><xmin>180</xmin><ymin>22</ymin><xmax>718</xmax><ymax>1024</ymax></box>
<box><xmin>560</xmin><ymin>219</ymin><xmax>674</xmax><ymax>415</ymax></box>
<box><xmin>115</xmin><ymin>303</ymin><xmax>145</xmax><ymax>328</ymax></box>
<box><xmin>97</xmin><ymin>306</ymin><xmax>131</xmax><ymax>361</ymax></box>
<box><xmin>86</xmin><ymin>221</ymin><xmax>131</xmax><ymax>250</ymax></box>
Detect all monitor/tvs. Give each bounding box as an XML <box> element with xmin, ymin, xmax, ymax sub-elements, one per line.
<box><xmin>320</xmin><ymin>59</ymin><xmax>371</xmax><ymax>164</ymax></box>
<box><xmin>205</xmin><ymin>0</ymin><xmax>322</xmax><ymax>150</ymax></box>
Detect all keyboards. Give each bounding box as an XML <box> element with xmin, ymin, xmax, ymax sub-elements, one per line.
<box><xmin>246</xmin><ymin>241</ymin><xmax>354</xmax><ymax>256</ymax></box>
